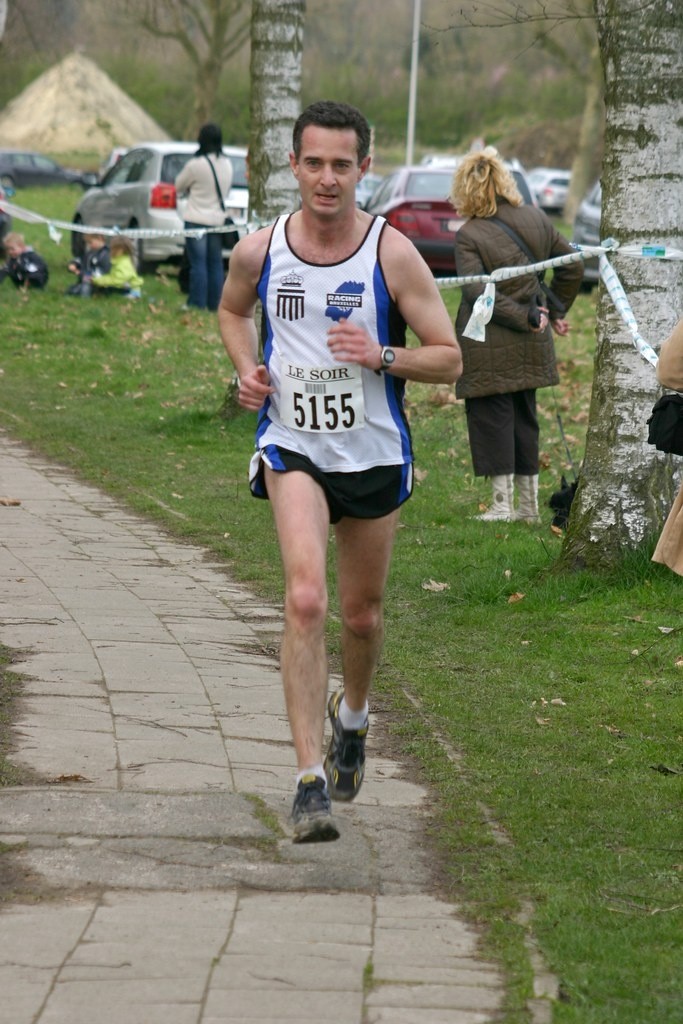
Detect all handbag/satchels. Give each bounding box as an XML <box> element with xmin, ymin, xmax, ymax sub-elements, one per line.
<box><xmin>222</xmin><ymin>218</ymin><xmax>240</xmax><ymax>248</ymax></box>
<box><xmin>645</xmin><ymin>392</ymin><xmax>683</xmax><ymax>457</ymax></box>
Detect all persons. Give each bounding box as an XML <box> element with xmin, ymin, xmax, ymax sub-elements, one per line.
<box><xmin>448</xmin><ymin>151</ymin><xmax>584</xmax><ymax>523</ymax></box>
<box><xmin>175</xmin><ymin>124</ymin><xmax>233</xmax><ymax>313</ymax></box>
<box><xmin>0</xmin><ymin>232</ymin><xmax>49</xmax><ymax>288</ymax></box>
<box><xmin>67</xmin><ymin>224</ymin><xmax>144</xmax><ymax>300</ymax></box>
<box><xmin>651</xmin><ymin>316</ymin><xmax>683</xmax><ymax>577</ymax></box>
<box><xmin>219</xmin><ymin>101</ymin><xmax>464</xmax><ymax>844</ymax></box>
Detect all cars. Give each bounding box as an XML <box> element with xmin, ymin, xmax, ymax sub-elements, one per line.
<box><xmin>0</xmin><ymin>150</ymin><xmax>99</xmax><ymax>195</ymax></box>
<box><xmin>353</xmin><ymin>154</ymin><xmax>602</xmax><ymax>294</ymax></box>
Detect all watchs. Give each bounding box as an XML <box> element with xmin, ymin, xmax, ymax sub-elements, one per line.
<box><xmin>374</xmin><ymin>345</ymin><xmax>396</xmax><ymax>377</ymax></box>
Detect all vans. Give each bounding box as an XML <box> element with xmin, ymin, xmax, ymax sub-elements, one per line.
<box><xmin>69</xmin><ymin>141</ymin><xmax>251</xmax><ymax>277</ymax></box>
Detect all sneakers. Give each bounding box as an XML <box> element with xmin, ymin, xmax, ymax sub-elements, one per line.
<box><xmin>290</xmin><ymin>776</ymin><xmax>341</xmax><ymax>843</ymax></box>
<box><xmin>323</xmin><ymin>689</ymin><xmax>369</xmax><ymax>801</ymax></box>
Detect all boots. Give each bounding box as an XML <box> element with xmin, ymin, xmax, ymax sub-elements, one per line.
<box><xmin>516</xmin><ymin>474</ymin><xmax>541</xmax><ymax>524</ymax></box>
<box><xmin>467</xmin><ymin>472</ymin><xmax>515</xmax><ymax>524</ymax></box>
